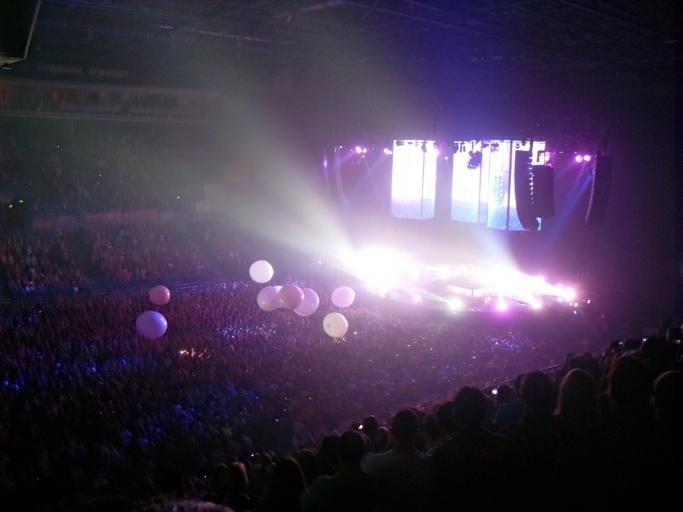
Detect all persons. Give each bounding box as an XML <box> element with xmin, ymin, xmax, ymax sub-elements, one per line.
<box><xmin>0</xmin><ymin>143</ymin><xmax>681</xmax><ymax>512</ymax></box>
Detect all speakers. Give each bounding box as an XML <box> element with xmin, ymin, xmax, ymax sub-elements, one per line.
<box><xmin>0</xmin><ymin>0</ymin><xmax>41</xmax><ymax>66</ymax></box>
<box><xmin>515</xmin><ymin>150</ymin><xmax>539</xmax><ymax>230</ymax></box>
<box><xmin>532</xmin><ymin>165</ymin><xmax>553</xmax><ymax>217</ymax></box>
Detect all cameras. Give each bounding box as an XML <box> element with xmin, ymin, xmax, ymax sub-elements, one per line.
<box><xmin>491</xmin><ymin>388</ymin><xmax>498</xmax><ymax>395</ymax></box>
<box><xmin>358</xmin><ymin>424</ymin><xmax>364</xmax><ymax>430</ymax></box>
